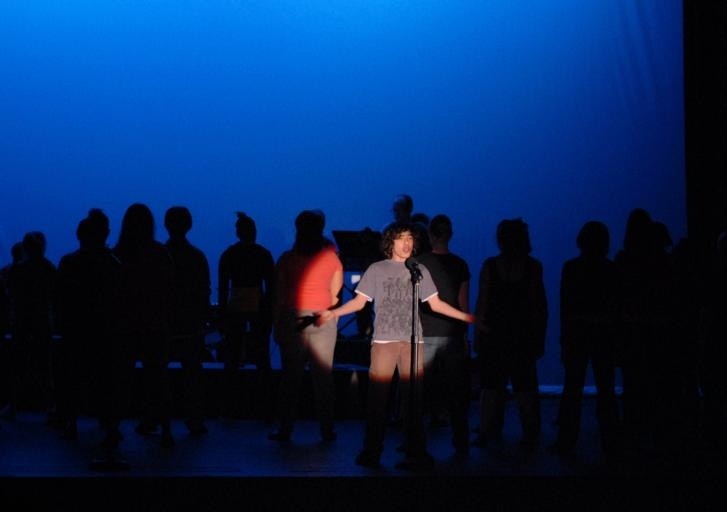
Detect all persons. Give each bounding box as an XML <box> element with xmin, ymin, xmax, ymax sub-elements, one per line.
<box><xmin>561</xmin><ymin>209</ymin><xmax>691</xmax><ymax>479</ymax></box>
<box><xmin>218</xmin><ymin>194</ymin><xmax>549</xmax><ymax>472</ymax></box>
<box><xmin>2</xmin><ymin>203</ymin><xmax>212</xmax><ymax>445</ymax></box>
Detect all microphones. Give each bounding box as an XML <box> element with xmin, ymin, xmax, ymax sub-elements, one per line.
<box><xmin>404</xmin><ymin>255</ymin><xmax>423</xmax><ymax>280</ymax></box>
